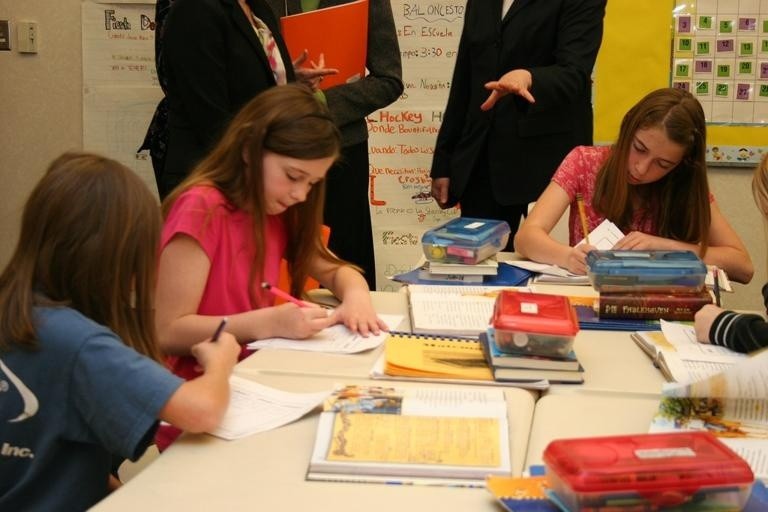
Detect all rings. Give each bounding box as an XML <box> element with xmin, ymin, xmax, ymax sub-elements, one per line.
<box><xmin>319</xmin><ymin>76</ymin><xmax>324</xmax><ymax>81</ymax></box>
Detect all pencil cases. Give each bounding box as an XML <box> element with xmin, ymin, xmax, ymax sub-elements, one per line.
<box><xmin>420</xmin><ymin>214</ymin><xmax>513</xmax><ymax>265</ymax></box>
<box><xmin>488</xmin><ymin>289</ymin><xmax>580</xmax><ymax>362</ymax></box>
<box><xmin>540</xmin><ymin>427</ymin><xmax>756</xmax><ymax>512</ymax></box>
<box><xmin>585</xmin><ymin>249</ymin><xmax>709</xmax><ymax>295</ymax></box>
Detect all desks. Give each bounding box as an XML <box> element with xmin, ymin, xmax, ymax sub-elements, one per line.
<box><xmin>84</xmin><ymin>288</ymin><xmax>767</xmax><ymax>512</ymax></box>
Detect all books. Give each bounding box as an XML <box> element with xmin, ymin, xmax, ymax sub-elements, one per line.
<box><xmin>479</xmin><ymin>331</ymin><xmax>585</xmax><ymax>384</ymax></box>
<box><xmin>487</xmin><ymin>326</ymin><xmax>580</xmax><ymax>371</ymax></box>
<box><xmin>308</xmin><ymin>384</ymin><xmax>513</xmax><ymax>489</ymax></box>
<box><xmin>406</xmin><ymin>284</ymin><xmax>529</xmax><ymax>338</ymax></box>
<box><xmin>417</xmin><ymin>261</ymin><xmax>484</xmax><ymax>284</ymax></box>
<box><xmin>533</xmin><ymin>218</ymin><xmax>733</xmax><ymax>293</ymax></box>
<box><xmin>598</xmin><ymin>295</ymin><xmax>713</xmax><ymax>321</ymax></box>
<box><xmin>385</xmin><ymin>331</ymin><xmax>550</xmax><ymax>392</ymax></box>
<box><xmin>282</xmin><ymin>2</ymin><xmax>368</xmax><ymax>96</ymax></box>
<box><xmin>428</xmin><ymin>255</ymin><xmax>498</xmax><ymax>277</ymax></box>
<box><xmin>631</xmin><ymin>320</ymin><xmax>767</xmax><ymax>400</ymax></box>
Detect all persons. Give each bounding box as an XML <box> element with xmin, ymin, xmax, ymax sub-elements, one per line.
<box><xmin>514</xmin><ymin>88</ymin><xmax>755</xmax><ymax>285</ymax></box>
<box><xmin>269</xmin><ymin>1</ymin><xmax>404</xmax><ymax>289</ymax></box>
<box><xmin>429</xmin><ymin>0</ymin><xmax>608</xmax><ymax>252</ymax></box>
<box><xmin>141</xmin><ymin>84</ymin><xmax>391</xmax><ymax>453</ymax></box>
<box><xmin>694</xmin><ymin>154</ymin><xmax>768</xmax><ymax>352</ymax></box>
<box><xmin>0</xmin><ymin>153</ymin><xmax>241</xmax><ymax>510</ymax></box>
<box><xmin>138</xmin><ymin>1</ymin><xmax>296</xmax><ymax>213</ymax></box>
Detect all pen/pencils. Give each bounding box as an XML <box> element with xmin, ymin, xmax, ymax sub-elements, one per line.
<box><xmin>574</xmin><ymin>191</ymin><xmax>589</xmax><ymax>246</ymax></box>
<box><xmin>712</xmin><ymin>268</ymin><xmax>723</xmax><ymax>310</ymax></box>
<box><xmin>209</xmin><ymin>315</ymin><xmax>227</xmax><ymax>340</ymax></box>
<box><xmin>309</xmin><ymin>76</ymin><xmax>324</xmax><ymax>92</ymax></box>
<box><xmin>258</xmin><ymin>279</ymin><xmax>310</xmax><ymax>308</ymax></box>
